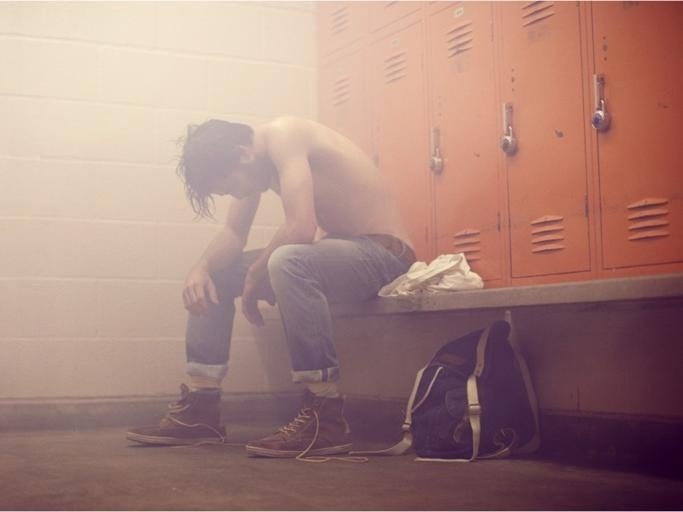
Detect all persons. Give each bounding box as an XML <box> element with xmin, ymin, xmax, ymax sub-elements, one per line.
<box><xmin>125</xmin><ymin>117</ymin><xmax>418</xmax><ymax>460</ymax></box>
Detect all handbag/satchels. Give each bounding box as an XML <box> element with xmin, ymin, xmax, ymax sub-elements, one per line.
<box><xmin>402</xmin><ymin>320</ymin><xmax>541</xmax><ymax>462</ymax></box>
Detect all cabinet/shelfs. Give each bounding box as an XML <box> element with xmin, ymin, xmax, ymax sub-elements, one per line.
<box><xmin>303</xmin><ymin>4</ymin><xmax>682</xmax><ymax>288</ymax></box>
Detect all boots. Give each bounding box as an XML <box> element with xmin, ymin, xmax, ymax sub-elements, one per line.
<box><xmin>246</xmin><ymin>388</ymin><xmax>352</xmax><ymax>458</ymax></box>
<box><xmin>126</xmin><ymin>383</ymin><xmax>228</xmax><ymax>447</ymax></box>
